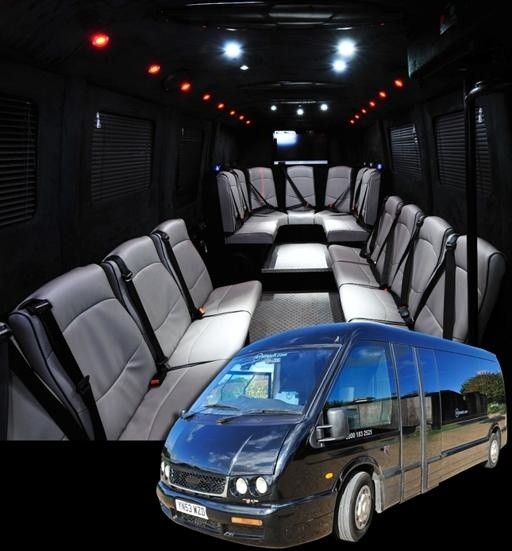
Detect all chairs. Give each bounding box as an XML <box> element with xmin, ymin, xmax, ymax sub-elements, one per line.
<box><xmin>1</xmin><ymin>165</ymin><xmax>507</xmax><ymax>444</ymax></box>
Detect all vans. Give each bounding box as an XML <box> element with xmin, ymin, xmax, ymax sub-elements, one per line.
<box><xmin>154</xmin><ymin>320</ymin><xmax>507</xmax><ymax>551</ymax></box>
